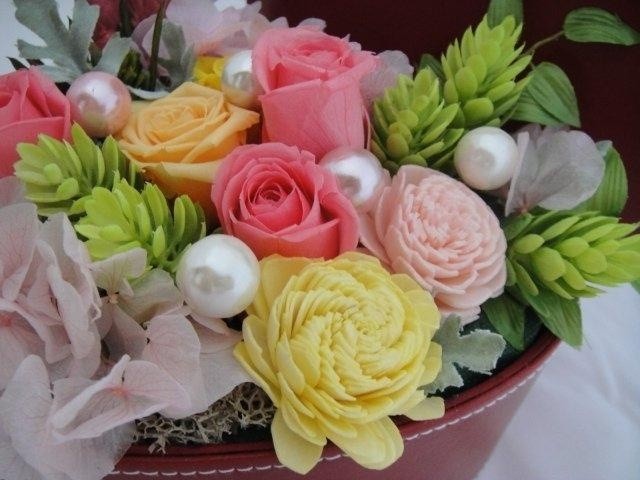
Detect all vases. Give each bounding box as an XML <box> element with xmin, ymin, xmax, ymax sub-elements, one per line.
<box><xmin>105</xmin><ymin>332</ymin><xmax>560</xmax><ymax>480</ymax></box>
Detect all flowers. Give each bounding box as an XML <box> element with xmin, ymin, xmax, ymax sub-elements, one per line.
<box><xmin>0</xmin><ymin>1</ymin><xmax>639</xmax><ymax>480</ymax></box>
